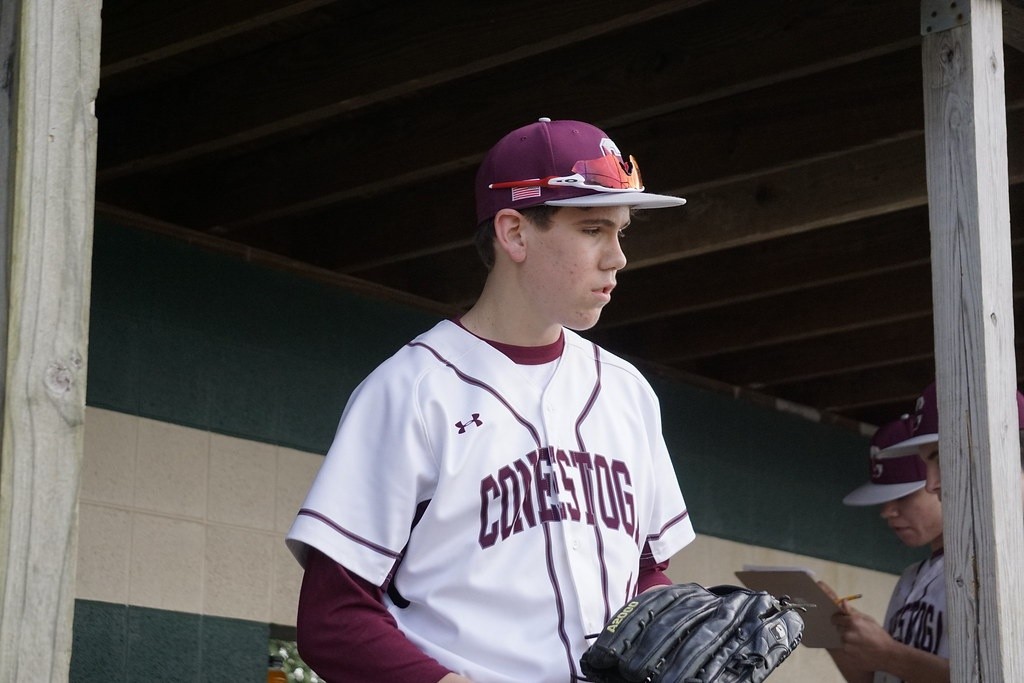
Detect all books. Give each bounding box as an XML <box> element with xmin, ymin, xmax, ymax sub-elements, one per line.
<box><xmin>735</xmin><ymin>564</ymin><xmax>854</xmax><ymax>649</ymax></box>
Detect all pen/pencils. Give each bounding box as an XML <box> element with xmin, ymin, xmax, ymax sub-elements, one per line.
<box><xmin>835</xmin><ymin>593</ymin><xmax>862</xmax><ymax>607</ymax></box>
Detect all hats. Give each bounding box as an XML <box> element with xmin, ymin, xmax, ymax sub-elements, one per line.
<box><xmin>475</xmin><ymin>117</ymin><xmax>686</xmax><ymax>226</ymax></box>
<box><xmin>876</xmin><ymin>383</ymin><xmax>1024</xmax><ymax>461</ymax></box>
<box><xmin>843</xmin><ymin>413</ymin><xmax>927</xmax><ymax>506</ymax></box>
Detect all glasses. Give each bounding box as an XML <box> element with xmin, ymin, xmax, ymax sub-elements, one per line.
<box><xmin>489</xmin><ymin>155</ymin><xmax>645</xmax><ymax>193</ymax></box>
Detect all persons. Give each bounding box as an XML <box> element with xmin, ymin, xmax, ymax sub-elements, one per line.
<box><xmin>283</xmin><ymin>117</ymin><xmax>696</xmax><ymax>683</ymax></box>
<box><xmin>816</xmin><ymin>386</ymin><xmax>1024</xmax><ymax>683</ymax></box>
